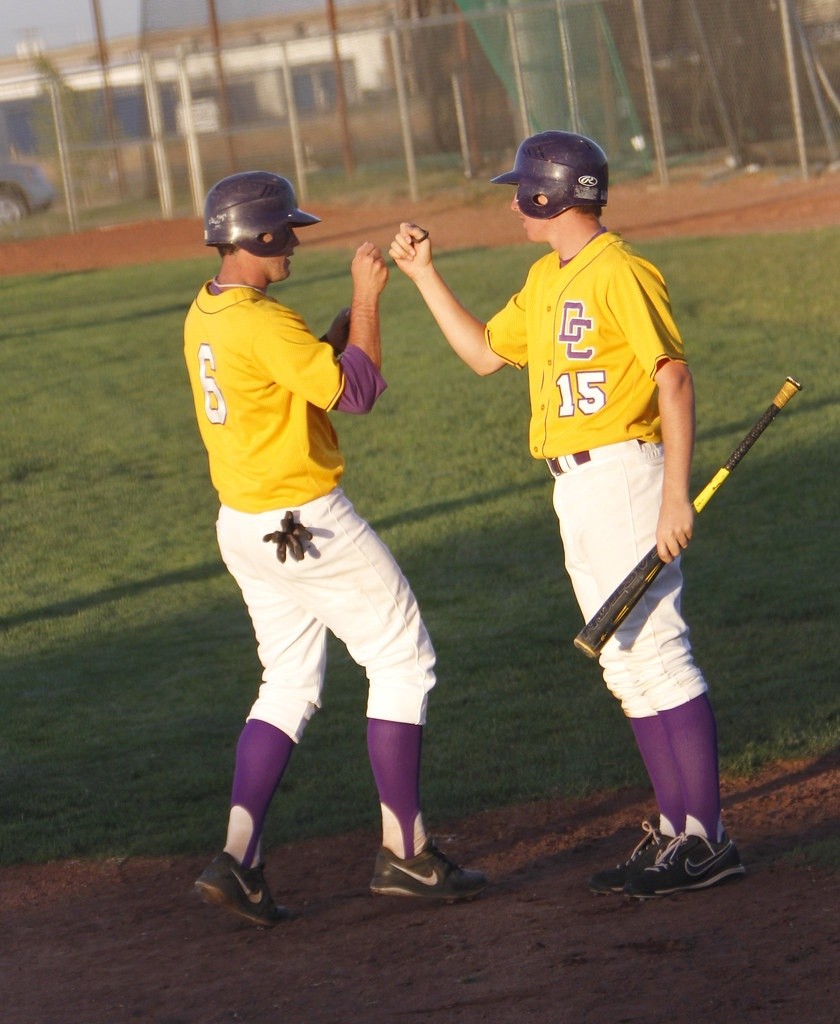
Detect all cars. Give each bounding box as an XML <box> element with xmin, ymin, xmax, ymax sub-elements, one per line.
<box><xmin>0</xmin><ymin>160</ymin><xmax>54</xmax><ymax>227</ymax></box>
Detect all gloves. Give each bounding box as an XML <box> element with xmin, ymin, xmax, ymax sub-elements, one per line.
<box><xmin>262</xmin><ymin>511</ymin><xmax>312</xmax><ymax>564</ymax></box>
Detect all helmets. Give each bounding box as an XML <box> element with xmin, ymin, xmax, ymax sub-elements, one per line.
<box><xmin>490</xmin><ymin>130</ymin><xmax>608</xmax><ymax>220</ymax></box>
<box><xmin>203</xmin><ymin>170</ymin><xmax>322</xmax><ymax>257</ymax></box>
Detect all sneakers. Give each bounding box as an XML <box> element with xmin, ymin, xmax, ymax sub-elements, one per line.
<box><xmin>370</xmin><ymin>831</ymin><xmax>488</xmax><ymax>904</ymax></box>
<box><xmin>587</xmin><ymin>821</ymin><xmax>677</xmax><ymax>897</ymax></box>
<box><xmin>195</xmin><ymin>851</ymin><xmax>295</xmax><ymax>926</ymax></box>
<box><xmin>622</xmin><ymin>830</ymin><xmax>745</xmax><ymax>898</ymax></box>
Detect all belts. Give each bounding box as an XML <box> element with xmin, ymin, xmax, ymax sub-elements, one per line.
<box><xmin>550</xmin><ymin>440</ymin><xmax>647</xmax><ymax>473</ymax></box>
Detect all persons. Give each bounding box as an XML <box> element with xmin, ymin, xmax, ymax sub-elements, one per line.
<box><xmin>389</xmin><ymin>131</ymin><xmax>747</xmax><ymax>897</ymax></box>
<box><xmin>184</xmin><ymin>171</ymin><xmax>497</xmax><ymax>928</ymax></box>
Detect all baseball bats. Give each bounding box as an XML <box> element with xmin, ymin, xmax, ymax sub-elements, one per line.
<box><xmin>574</xmin><ymin>376</ymin><xmax>803</xmax><ymax>658</ymax></box>
